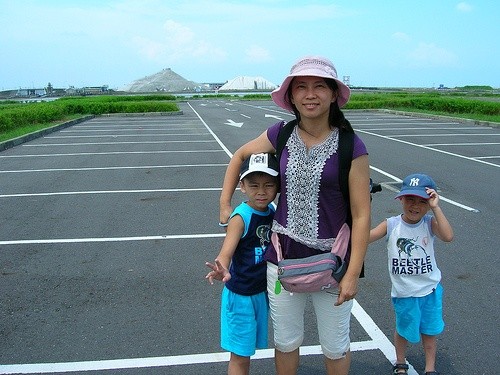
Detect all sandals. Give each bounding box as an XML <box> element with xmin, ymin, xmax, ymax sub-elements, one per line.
<box><xmin>424</xmin><ymin>371</ymin><xmax>436</xmax><ymax>375</ymax></box>
<box><xmin>393</xmin><ymin>364</ymin><xmax>409</xmax><ymax>375</ymax></box>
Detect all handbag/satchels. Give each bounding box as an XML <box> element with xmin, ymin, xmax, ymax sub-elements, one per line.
<box><xmin>268</xmin><ymin>216</ymin><xmax>352</xmax><ymax>293</ymax></box>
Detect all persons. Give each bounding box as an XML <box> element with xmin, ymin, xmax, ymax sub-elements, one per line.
<box><xmin>205</xmin><ymin>151</ymin><xmax>282</xmax><ymax>375</ymax></box>
<box><xmin>366</xmin><ymin>173</ymin><xmax>455</xmax><ymax>375</ymax></box>
<box><xmin>218</xmin><ymin>55</ymin><xmax>371</xmax><ymax>375</ymax></box>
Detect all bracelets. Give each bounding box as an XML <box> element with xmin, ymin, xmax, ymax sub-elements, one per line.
<box><xmin>218</xmin><ymin>222</ymin><xmax>230</xmax><ymax>227</ymax></box>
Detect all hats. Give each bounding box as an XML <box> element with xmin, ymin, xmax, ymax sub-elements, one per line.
<box><xmin>270</xmin><ymin>57</ymin><xmax>351</xmax><ymax>111</ymax></box>
<box><xmin>238</xmin><ymin>153</ymin><xmax>279</xmax><ymax>181</ymax></box>
<box><xmin>394</xmin><ymin>173</ymin><xmax>437</xmax><ymax>200</ymax></box>
